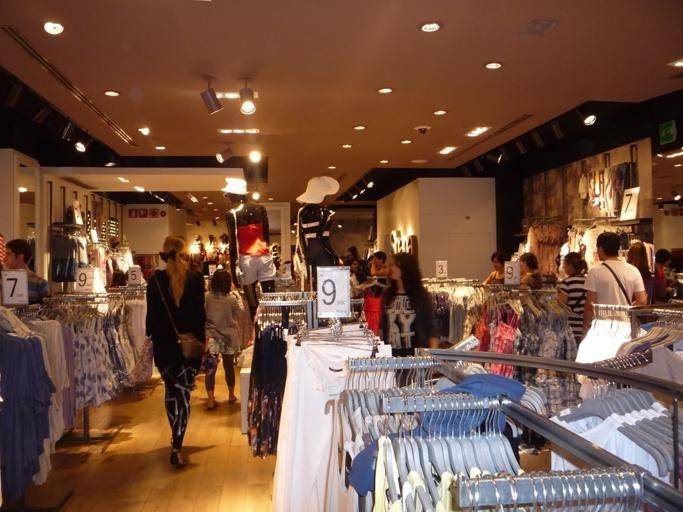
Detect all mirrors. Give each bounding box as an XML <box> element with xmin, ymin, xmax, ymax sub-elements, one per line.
<box><xmin>12</xmin><ymin>154</ymin><xmax>42</xmax><ymax>274</ymax></box>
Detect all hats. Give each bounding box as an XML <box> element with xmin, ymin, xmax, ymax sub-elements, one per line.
<box><xmin>295</xmin><ymin>174</ymin><xmax>341</xmax><ymax>207</ymax></box>
<box><xmin>219</xmin><ymin>177</ymin><xmax>251</xmax><ymax>196</ymax></box>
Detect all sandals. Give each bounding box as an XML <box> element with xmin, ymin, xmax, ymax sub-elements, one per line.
<box><xmin>170</xmin><ymin>448</ymin><xmax>187</xmax><ymax>469</ymax></box>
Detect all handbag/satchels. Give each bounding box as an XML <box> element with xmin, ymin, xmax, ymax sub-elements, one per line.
<box><xmin>177</xmin><ymin>330</ymin><xmax>205</xmax><ymax>360</ymax></box>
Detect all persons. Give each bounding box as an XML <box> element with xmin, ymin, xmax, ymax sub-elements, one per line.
<box><xmin>205</xmin><ymin>234</ymin><xmax>218</xmax><ymax>261</ymax></box>
<box><xmin>626</xmin><ymin>243</ymin><xmax>653</xmax><ymax>303</ymax></box>
<box><xmin>582</xmin><ymin>232</ymin><xmax>648</xmax><ymax>338</ymax></box>
<box><xmin>654</xmin><ymin>249</ymin><xmax>674</xmax><ymax>301</ymax></box>
<box><xmin>220</xmin><ymin>178</ymin><xmax>275</xmax><ymax>326</ymax></box>
<box><xmin>146</xmin><ymin>234</ymin><xmax>206</xmax><ymax>470</ymax></box>
<box><xmin>63</xmin><ymin>194</ymin><xmax>89</xmax><ymax>226</ymax></box>
<box><xmin>347</xmin><ymin>246</ymin><xmax>366</xmax><ymax>270</ymax></box>
<box><xmin>380</xmin><ymin>253</ymin><xmax>439</xmax><ymax>385</ymax></box>
<box><xmin>369</xmin><ymin>251</ymin><xmax>388</xmax><ymax>277</ymax></box>
<box><xmin>558</xmin><ymin>252</ymin><xmax>587</xmax><ymax>340</ymax></box>
<box><xmin>347</xmin><ymin>257</ymin><xmax>374</xmax><ymax>298</ymax></box>
<box><xmin>190</xmin><ymin>236</ymin><xmax>205</xmax><ymax>261</ymax></box>
<box><xmin>3</xmin><ymin>239</ymin><xmax>51</xmax><ymax>302</ymax></box>
<box><xmin>519</xmin><ymin>253</ymin><xmax>542</xmax><ymax>290</ymax></box>
<box><xmin>483</xmin><ymin>252</ymin><xmax>506</xmax><ymax>284</ymax></box>
<box><xmin>296</xmin><ymin>175</ymin><xmax>344</xmax><ymax>287</ymax></box>
<box><xmin>205</xmin><ymin>269</ymin><xmax>244</xmax><ymax>408</ymax></box>
<box><xmin>218</xmin><ymin>234</ymin><xmax>232</xmax><ymax>263</ymax></box>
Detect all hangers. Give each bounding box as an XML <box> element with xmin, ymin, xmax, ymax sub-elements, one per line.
<box><xmin>6</xmin><ymin>285</ymin><xmax>146</xmax><ymax>325</ymax></box>
<box><xmin>525</xmin><ymin>215</ymin><xmax>609</xmax><ymax>232</ymax></box>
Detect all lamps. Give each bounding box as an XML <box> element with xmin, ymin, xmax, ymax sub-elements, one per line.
<box><xmin>198</xmin><ymin>76</ymin><xmax>223</xmax><ymax>115</ymax></box>
<box><xmin>485</xmin><ymin>151</ymin><xmax>505</xmax><ymax>163</ymax></box>
<box><xmin>3</xmin><ymin>83</ymin><xmax>22</xmax><ymax>107</ymax></box>
<box><xmin>30</xmin><ymin>106</ymin><xmax>53</xmax><ymax>124</ymax></box>
<box><xmin>574</xmin><ymin>102</ymin><xmax>599</xmax><ymax>131</ymax></box>
<box><xmin>72</xmin><ymin>130</ymin><xmax>94</xmax><ymax>155</ymax></box>
<box><xmin>56</xmin><ymin>120</ymin><xmax>74</xmax><ymax>145</ymax></box>
<box><xmin>215</xmin><ymin>143</ymin><xmax>234</xmax><ymax>164</ymax></box>
<box><xmin>237</xmin><ymin>77</ymin><xmax>258</xmax><ymax>116</ymax></box>
<box><xmin>104</xmin><ymin>147</ymin><xmax>117</xmax><ymax>167</ymax></box>
